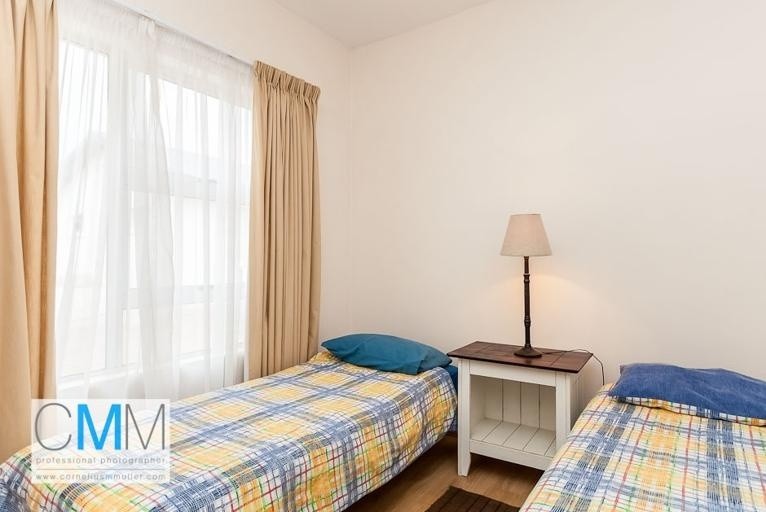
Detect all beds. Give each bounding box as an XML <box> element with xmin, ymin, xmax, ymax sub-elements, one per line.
<box><xmin>521</xmin><ymin>383</ymin><xmax>766</xmax><ymax>512</ymax></box>
<box><xmin>2</xmin><ymin>355</ymin><xmax>458</xmax><ymax>511</ymax></box>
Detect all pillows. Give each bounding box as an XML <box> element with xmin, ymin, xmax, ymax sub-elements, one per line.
<box><xmin>321</xmin><ymin>332</ymin><xmax>452</xmax><ymax>375</ymax></box>
<box><xmin>609</xmin><ymin>363</ymin><xmax>766</xmax><ymax>429</ymax></box>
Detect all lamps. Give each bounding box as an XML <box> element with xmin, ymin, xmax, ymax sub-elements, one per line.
<box><xmin>500</xmin><ymin>214</ymin><xmax>553</xmax><ymax>358</ymax></box>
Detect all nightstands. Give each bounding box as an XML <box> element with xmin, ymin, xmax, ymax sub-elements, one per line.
<box><xmin>449</xmin><ymin>340</ymin><xmax>595</xmax><ymax>478</ymax></box>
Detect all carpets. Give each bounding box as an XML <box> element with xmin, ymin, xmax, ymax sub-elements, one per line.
<box><xmin>426</xmin><ymin>485</ymin><xmax>521</xmax><ymax>512</ymax></box>
